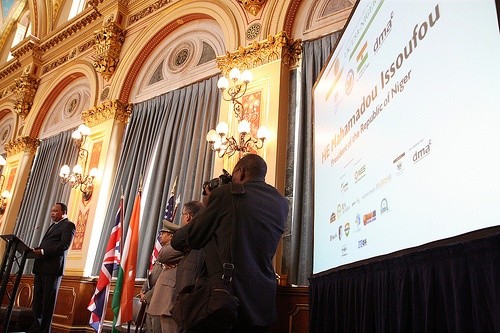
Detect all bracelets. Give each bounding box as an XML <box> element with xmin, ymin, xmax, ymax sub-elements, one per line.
<box><xmin>40</xmin><ymin>249</ymin><xmax>41</xmax><ymax>256</ymax></box>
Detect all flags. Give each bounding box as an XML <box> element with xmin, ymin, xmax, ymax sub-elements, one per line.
<box><xmin>111</xmin><ymin>190</ymin><xmax>142</xmax><ymax>333</ymax></box>
<box><xmin>148</xmin><ymin>185</ymin><xmax>179</xmax><ymax>271</ymax></box>
<box><xmin>87</xmin><ymin>195</ymin><xmax>125</xmax><ymax>333</ymax></box>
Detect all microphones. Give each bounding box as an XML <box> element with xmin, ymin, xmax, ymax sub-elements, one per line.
<box><xmin>17</xmin><ymin>227</ymin><xmax>39</xmax><ymax>237</ymax></box>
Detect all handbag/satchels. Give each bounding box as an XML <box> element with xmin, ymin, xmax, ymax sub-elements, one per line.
<box><xmin>172</xmin><ymin>284</ymin><xmax>240</xmax><ymax>333</ymax></box>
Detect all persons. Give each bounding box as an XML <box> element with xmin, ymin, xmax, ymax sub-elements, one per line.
<box><xmin>26</xmin><ymin>202</ymin><xmax>76</xmax><ymax>333</ymax></box>
<box><xmin>156</xmin><ymin>201</ymin><xmax>206</xmax><ymax>333</ymax></box>
<box><xmin>170</xmin><ymin>154</ymin><xmax>290</xmax><ymax>333</ymax></box>
<box><xmin>139</xmin><ymin>219</ymin><xmax>183</xmax><ymax>333</ymax></box>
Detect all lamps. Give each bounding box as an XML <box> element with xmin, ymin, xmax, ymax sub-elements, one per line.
<box><xmin>217</xmin><ymin>67</ymin><xmax>250</xmax><ymax>121</ymax></box>
<box><xmin>60</xmin><ymin>164</ymin><xmax>97</xmax><ymax>201</ymax></box>
<box><xmin>206</xmin><ymin>118</ymin><xmax>269</xmax><ymax>161</ymax></box>
<box><xmin>72</xmin><ymin>123</ymin><xmax>92</xmax><ymax>165</ymax></box>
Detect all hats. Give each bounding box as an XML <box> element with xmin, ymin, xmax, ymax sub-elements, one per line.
<box><xmin>158</xmin><ymin>219</ymin><xmax>182</xmax><ymax>233</ymax></box>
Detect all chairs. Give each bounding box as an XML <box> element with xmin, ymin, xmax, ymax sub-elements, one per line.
<box><xmin>127</xmin><ymin>294</ymin><xmax>148</xmax><ymax>333</ymax></box>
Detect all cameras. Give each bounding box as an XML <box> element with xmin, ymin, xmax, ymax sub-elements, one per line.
<box><xmin>202</xmin><ymin>169</ymin><xmax>231</xmax><ymax>195</ymax></box>
